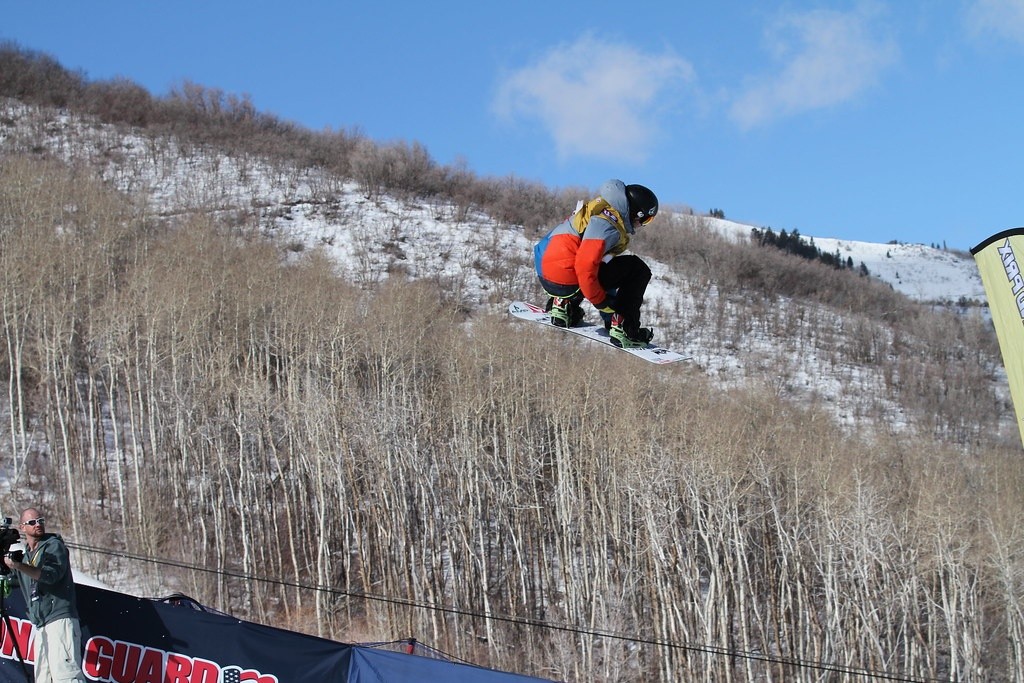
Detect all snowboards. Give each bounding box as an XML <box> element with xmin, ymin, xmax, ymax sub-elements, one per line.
<box><xmin>508</xmin><ymin>301</ymin><xmax>693</xmax><ymax>367</ymax></box>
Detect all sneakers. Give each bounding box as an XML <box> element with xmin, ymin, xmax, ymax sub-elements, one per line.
<box><xmin>611</xmin><ymin>327</ymin><xmax>653</xmax><ymax>348</ymax></box>
<box><xmin>551</xmin><ymin>305</ymin><xmax>585</xmax><ymax>327</ymax></box>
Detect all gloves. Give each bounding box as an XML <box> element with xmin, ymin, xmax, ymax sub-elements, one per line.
<box><xmin>594</xmin><ymin>296</ymin><xmax>618</xmax><ymax>319</ymax></box>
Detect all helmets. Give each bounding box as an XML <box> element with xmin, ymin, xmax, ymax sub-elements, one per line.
<box><xmin>625</xmin><ymin>184</ymin><xmax>658</xmax><ymax>223</ymax></box>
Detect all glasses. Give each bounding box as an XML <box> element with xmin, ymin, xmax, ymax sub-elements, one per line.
<box><xmin>23</xmin><ymin>518</ymin><xmax>44</xmax><ymax>526</ymax></box>
<box><xmin>640</xmin><ymin>217</ymin><xmax>653</xmax><ymax>226</ymax></box>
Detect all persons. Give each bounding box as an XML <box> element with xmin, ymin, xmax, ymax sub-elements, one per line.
<box><xmin>534</xmin><ymin>179</ymin><xmax>658</xmax><ymax>347</ymax></box>
<box><xmin>4</xmin><ymin>508</ymin><xmax>86</xmax><ymax>683</ymax></box>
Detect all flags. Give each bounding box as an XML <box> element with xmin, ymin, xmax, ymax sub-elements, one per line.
<box><xmin>971</xmin><ymin>226</ymin><xmax>1024</xmax><ymax>447</ymax></box>
